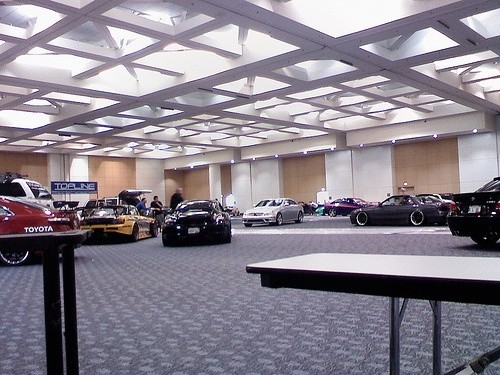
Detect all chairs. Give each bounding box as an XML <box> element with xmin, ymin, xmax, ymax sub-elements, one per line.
<box><xmin>349</xmin><ymin>200</ymin><xmax>354</xmax><ymax>204</ymax></box>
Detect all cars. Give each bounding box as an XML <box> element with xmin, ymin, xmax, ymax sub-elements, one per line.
<box><xmin>349</xmin><ymin>193</ymin><xmax>457</xmax><ymax>227</ymax></box>
<box><xmin>446</xmin><ymin>177</ymin><xmax>500</xmax><ymax>249</ymax></box>
<box><xmin>322</xmin><ymin>198</ymin><xmax>379</xmax><ymax>218</ymax></box>
<box><xmin>0</xmin><ymin>170</ymin><xmax>162</xmax><ymax>265</ymax></box>
<box><xmin>241</xmin><ymin>197</ymin><xmax>305</xmax><ymax>227</ymax></box>
<box><xmin>223</xmin><ymin>205</ymin><xmax>240</xmax><ymax>217</ymax></box>
<box><xmin>159</xmin><ymin>199</ymin><xmax>232</xmax><ymax>246</ymax></box>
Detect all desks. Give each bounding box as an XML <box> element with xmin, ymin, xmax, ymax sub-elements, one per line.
<box><xmin>246</xmin><ymin>253</ymin><xmax>500</xmax><ymax>375</ymax></box>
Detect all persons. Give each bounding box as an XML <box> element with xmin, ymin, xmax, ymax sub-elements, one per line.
<box><xmin>170</xmin><ymin>186</ymin><xmax>185</xmax><ymax>209</ymax></box>
<box><xmin>233</xmin><ymin>202</ymin><xmax>237</xmax><ymax>208</ymax></box>
<box><xmin>150</xmin><ymin>195</ymin><xmax>163</xmax><ymax>225</ymax></box>
<box><xmin>309</xmin><ymin>201</ymin><xmax>319</xmax><ymax>214</ymax></box>
<box><xmin>135</xmin><ymin>197</ymin><xmax>151</xmax><ymax>216</ymax></box>
<box><xmin>327</xmin><ymin>196</ymin><xmax>334</xmax><ymax>203</ymax></box>
